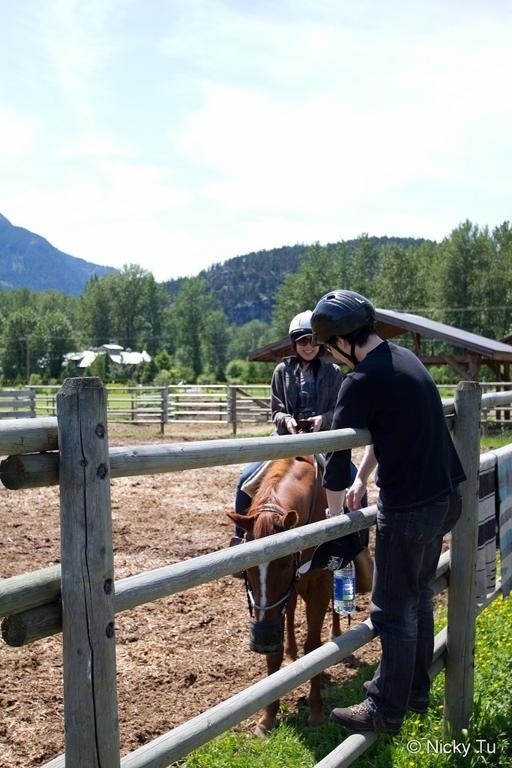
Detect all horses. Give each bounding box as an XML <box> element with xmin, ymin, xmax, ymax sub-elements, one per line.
<box><xmin>225</xmin><ymin>428</ymin><xmax>361</xmax><ymax>739</ymax></box>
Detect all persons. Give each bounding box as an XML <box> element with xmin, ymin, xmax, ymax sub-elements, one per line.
<box><xmin>309</xmin><ymin>289</ymin><xmax>469</xmax><ymax>734</ymax></box>
<box><xmin>234</xmin><ymin>309</ymin><xmax>370</xmax><ymax>551</ymax></box>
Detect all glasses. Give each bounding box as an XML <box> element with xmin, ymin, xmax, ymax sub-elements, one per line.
<box><xmin>298</xmin><ymin>338</ymin><xmax>314</xmax><ymax>346</ymax></box>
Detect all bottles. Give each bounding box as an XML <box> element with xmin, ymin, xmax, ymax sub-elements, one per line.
<box><xmin>333</xmin><ymin>560</ymin><xmax>356</xmax><ymax>615</ymax></box>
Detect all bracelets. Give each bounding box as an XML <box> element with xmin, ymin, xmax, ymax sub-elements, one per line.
<box><xmin>324</xmin><ymin>507</ymin><xmax>345</xmax><ymax>520</ymax></box>
<box><xmin>285</xmin><ymin>416</ymin><xmax>292</xmax><ymax>423</ymax></box>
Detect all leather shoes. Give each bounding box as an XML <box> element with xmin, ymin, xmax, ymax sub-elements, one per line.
<box><xmin>330</xmin><ymin>681</ymin><xmax>428</xmax><ymax>734</ymax></box>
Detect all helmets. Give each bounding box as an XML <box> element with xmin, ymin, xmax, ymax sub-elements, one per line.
<box><xmin>288</xmin><ymin>310</ymin><xmax>314</xmax><ymax>341</ymax></box>
<box><xmin>310</xmin><ymin>289</ymin><xmax>375</xmax><ymax>346</ymax></box>
